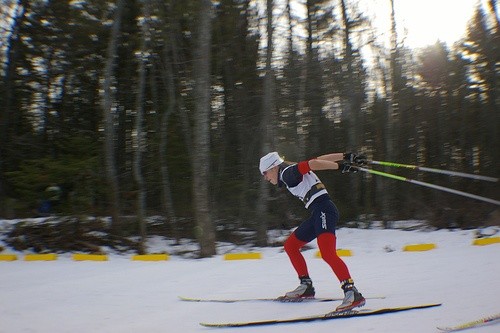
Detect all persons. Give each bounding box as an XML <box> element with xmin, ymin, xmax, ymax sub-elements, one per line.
<box><xmin>259</xmin><ymin>151</ymin><xmax>373</xmax><ymax>313</ymax></box>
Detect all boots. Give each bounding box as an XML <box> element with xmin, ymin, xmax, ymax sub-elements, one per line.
<box><xmin>285</xmin><ymin>275</ymin><xmax>317</xmax><ymax>301</ymax></box>
<box><xmin>335</xmin><ymin>278</ymin><xmax>367</xmax><ymax>312</ymax></box>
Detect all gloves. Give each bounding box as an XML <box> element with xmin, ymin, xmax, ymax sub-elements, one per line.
<box><xmin>339</xmin><ymin>164</ymin><xmax>363</xmax><ymax>176</ymax></box>
<box><xmin>342</xmin><ymin>152</ymin><xmax>369</xmax><ymax>167</ymax></box>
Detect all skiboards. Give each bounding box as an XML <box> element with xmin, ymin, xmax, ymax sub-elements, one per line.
<box><xmin>435</xmin><ymin>313</ymin><xmax>500</xmax><ymax>332</ymax></box>
<box><xmin>177</xmin><ymin>295</ymin><xmax>443</xmax><ymax>328</ymax></box>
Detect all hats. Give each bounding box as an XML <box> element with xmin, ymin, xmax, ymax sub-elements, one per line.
<box><xmin>259</xmin><ymin>152</ymin><xmax>284</xmax><ymax>175</ymax></box>
